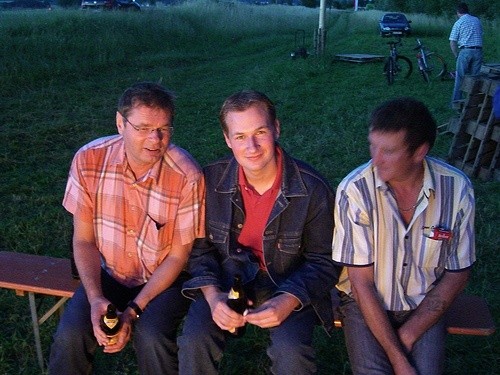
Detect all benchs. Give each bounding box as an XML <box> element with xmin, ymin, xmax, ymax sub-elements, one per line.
<box><xmin>0</xmin><ymin>250</ymin><xmax>497</xmax><ymax>375</ymax></box>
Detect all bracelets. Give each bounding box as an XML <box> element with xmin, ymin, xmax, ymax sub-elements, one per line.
<box><xmin>126</xmin><ymin>302</ymin><xmax>143</xmax><ymax>318</ymax></box>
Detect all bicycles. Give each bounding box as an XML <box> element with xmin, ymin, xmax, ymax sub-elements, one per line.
<box><xmin>413</xmin><ymin>36</ymin><xmax>447</xmax><ymax>85</ymax></box>
<box><xmin>383</xmin><ymin>37</ymin><xmax>414</xmax><ymax>86</ymax></box>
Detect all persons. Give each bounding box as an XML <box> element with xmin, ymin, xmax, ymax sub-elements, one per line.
<box><xmin>449</xmin><ymin>4</ymin><xmax>484</xmax><ymax>100</ymax></box>
<box><xmin>49</xmin><ymin>83</ymin><xmax>207</xmax><ymax>375</ymax></box>
<box><xmin>175</xmin><ymin>91</ymin><xmax>344</xmax><ymax>375</ymax></box>
<box><xmin>332</xmin><ymin>95</ymin><xmax>476</xmax><ymax>375</ymax></box>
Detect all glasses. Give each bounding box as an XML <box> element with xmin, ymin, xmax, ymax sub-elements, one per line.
<box><xmin>121</xmin><ymin>113</ymin><xmax>175</xmax><ymax>135</ymax></box>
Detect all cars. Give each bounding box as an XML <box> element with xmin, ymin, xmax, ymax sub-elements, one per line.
<box><xmin>379</xmin><ymin>12</ymin><xmax>412</xmax><ymax>36</ymax></box>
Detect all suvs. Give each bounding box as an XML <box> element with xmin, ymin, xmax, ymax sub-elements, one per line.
<box><xmin>81</xmin><ymin>0</ymin><xmax>142</xmax><ymax>13</ymax></box>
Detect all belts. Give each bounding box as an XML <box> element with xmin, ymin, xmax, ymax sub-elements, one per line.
<box><xmin>469</xmin><ymin>47</ymin><xmax>482</xmax><ymax>49</ymax></box>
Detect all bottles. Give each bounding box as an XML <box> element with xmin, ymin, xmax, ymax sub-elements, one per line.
<box><xmin>96</xmin><ymin>303</ymin><xmax>121</xmax><ymax>357</ymax></box>
<box><xmin>225</xmin><ymin>275</ymin><xmax>250</xmax><ymax>339</ymax></box>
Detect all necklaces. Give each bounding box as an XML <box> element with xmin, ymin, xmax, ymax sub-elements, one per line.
<box><xmin>399</xmin><ymin>206</ymin><xmax>415</xmax><ymax>212</ymax></box>
